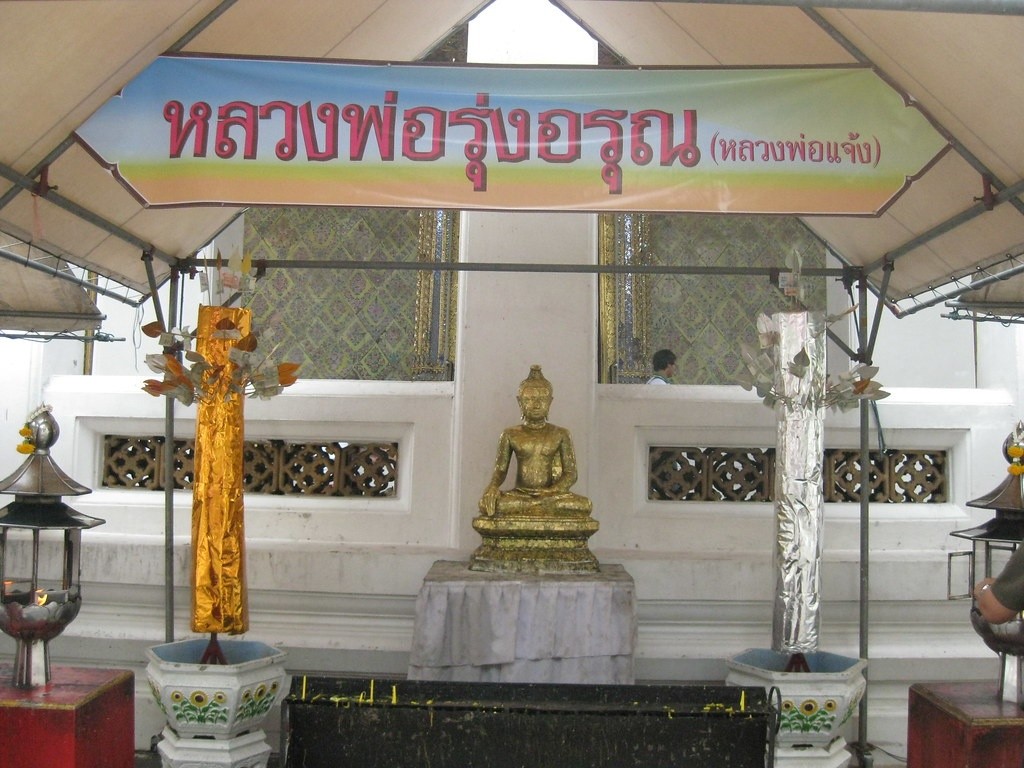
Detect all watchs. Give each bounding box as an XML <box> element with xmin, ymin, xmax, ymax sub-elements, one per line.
<box><xmin>978</xmin><ymin>584</ymin><xmax>991</xmax><ymax>595</ymax></box>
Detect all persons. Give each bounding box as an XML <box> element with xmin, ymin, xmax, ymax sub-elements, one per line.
<box><xmin>645</xmin><ymin>350</ymin><xmax>681</xmax><ymax>499</ymax></box>
<box><xmin>476</xmin><ymin>364</ymin><xmax>593</xmax><ymax>518</ymax></box>
<box><xmin>973</xmin><ymin>539</ymin><xmax>1024</xmax><ymax>625</ymax></box>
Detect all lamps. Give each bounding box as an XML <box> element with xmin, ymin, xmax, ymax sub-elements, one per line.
<box><xmin>949</xmin><ymin>424</ymin><xmax>1024</xmax><ymax>703</ymax></box>
<box><xmin>0</xmin><ymin>408</ymin><xmax>107</xmax><ymax>688</ymax></box>
